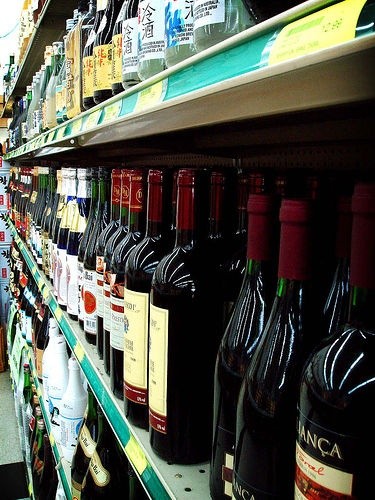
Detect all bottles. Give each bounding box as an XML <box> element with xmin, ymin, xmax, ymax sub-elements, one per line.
<box><xmin>4</xmin><ymin>164</ymin><xmax>375</xmax><ymax>500</ymax></box>
<box><xmin>2</xmin><ymin>0</ymin><xmax>255</xmax><ymax>154</ymax></box>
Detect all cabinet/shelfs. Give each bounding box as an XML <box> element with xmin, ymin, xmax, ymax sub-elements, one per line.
<box><xmin>3</xmin><ymin>0</ymin><xmax>374</xmax><ymax>500</ymax></box>
